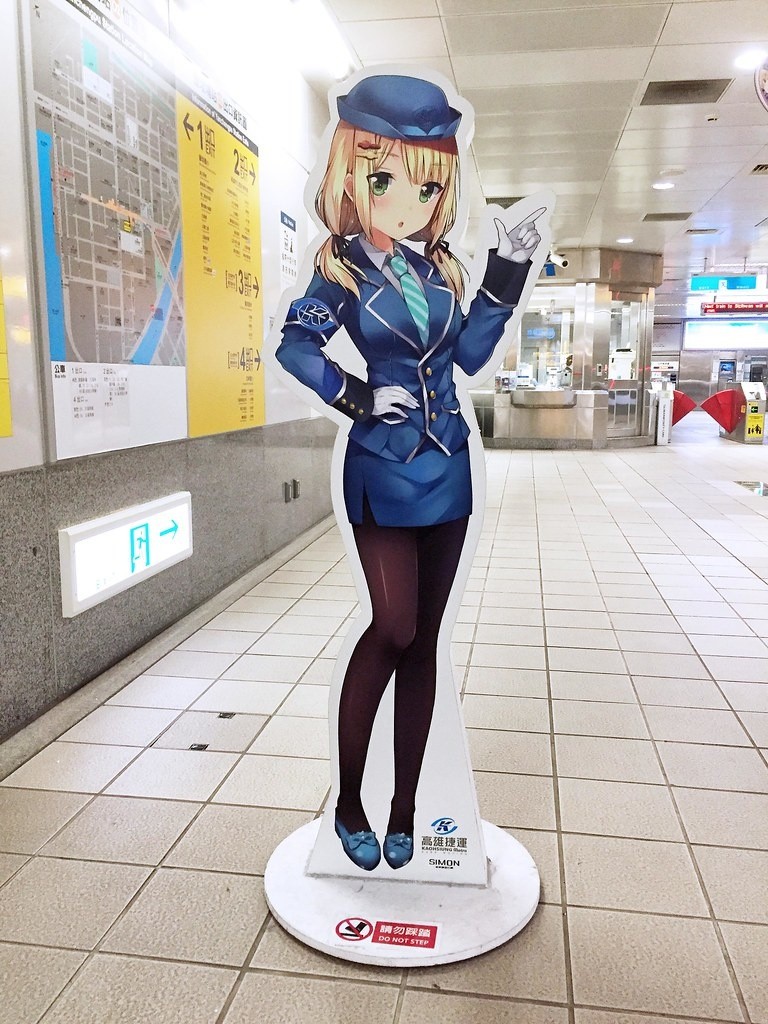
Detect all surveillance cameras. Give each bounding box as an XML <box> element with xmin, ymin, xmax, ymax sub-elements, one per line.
<box><xmin>549</xmin><ymin>253</ymin><xmax>569</xmax><ymax>268</ymax></box>
